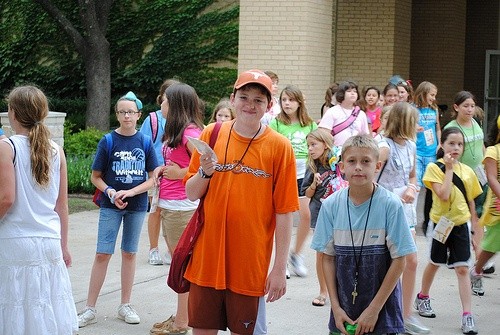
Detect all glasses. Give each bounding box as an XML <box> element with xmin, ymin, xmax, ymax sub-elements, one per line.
<box><xmin>115</xmin><ymin>109</ymin><xmax>142</xmax><ymax>116</ymax></box>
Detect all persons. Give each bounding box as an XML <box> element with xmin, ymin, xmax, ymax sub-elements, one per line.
<box><xmin>322</xmin><ymin>136</ymin><xmax>407</xmax><ymax>335</ymax></box>
<box><xmin>0</xmin><ymin>85</ymin><xmax>72</xmax><ymax>335</ymax></box>
<box><xmin>140</xmin><ymin>80</ymin><xmax>182</xmax><ymax>266</ymax></box>
<box><xmin>185</xmin><ymin>69</ymin><xmax>294</xmax><ymax>335</ymax></box>
<box><xmin>210</xmin><ymin>71</ymin><xmax>500</xmax><ymax>335</ymax></box>
<box><xmin>77</xmin><ymin>91</ymin><xmax>161</xmax><ymax>329</ymax></box>
<box><xmin>151</xmin><ymin>83</ymin><xmax>204</xmax><ymax>335</ymax></box>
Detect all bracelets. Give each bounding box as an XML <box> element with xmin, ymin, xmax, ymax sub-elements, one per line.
<box><xmin>111</xmin><ymin>194</ymin><xmax>116</xmax><ymax>204</ymax></box>
<box><xmin>310</xmin><ymin>186</ymin><xmax>315</xmax><ymax>191</ymax></box>
<box><xmin>104</xmin><ymin>186</ymin><xmax>112</xmax><ymax>196</ymax></box>
<box><xmin>409</xmin><ymin>186</ymin><xmax>416</xmax><ymax>193</ymax></box>
<box><xmin>409</xmin><ymin>183</ymin><xmax>417</xmax><ymax>191</ymax></box>
<box><xmin>108</xmin><ymin>189</ymin><xmax>116</xmax><ymax>199</ymax></box>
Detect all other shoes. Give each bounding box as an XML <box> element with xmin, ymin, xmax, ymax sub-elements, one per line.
<box><xmin>289</xmin><ymin>249</ymin><xmax>308</xmax><ymax>278</ymax></box>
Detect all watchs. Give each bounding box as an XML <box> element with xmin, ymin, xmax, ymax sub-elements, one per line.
<box><xmin>199</xmin><ymin>165</ymin><xmax>213</xmax><ymax>179</ymax></box>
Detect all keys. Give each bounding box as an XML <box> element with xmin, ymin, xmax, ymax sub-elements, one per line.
<box><xmin>352</xmin><ymin>291</ymin><xmax>358</xmax><ymax>304</ymax></box>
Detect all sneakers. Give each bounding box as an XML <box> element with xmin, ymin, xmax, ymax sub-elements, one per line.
<box><xmin>469</xmin><ymin>266</ymin><xmax>485</xmax><ymax>296</ymax></box>
<box><xmin>148</xmin><ymin>248</ymin><xmax>164</xmax><ymax>265</ymax></box>
<box><xmin>77</xmin><ymin>309</ymin><xmax>97</xmax><ymax>327</ymax></box>
<box><xmin>149</xmin><ymin>316</ymin><xmax>190</xmax><ymax>335</ymax></box>
<box><xmin>413</xmin><ymin>293</ymin><xmax>436</xmax><ymax>318</ymax></box>
<box><xmin>118</xmin><ymin>304</ymin><xmax>141</xmax><ymax>325</ymax></box>
<box><xmin>460</xmin><ymin>314</ymin><xmax>478</xmax><ymax>335</ymax></box>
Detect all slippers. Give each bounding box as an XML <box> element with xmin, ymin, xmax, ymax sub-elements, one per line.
<box><xmin>311</xmin><ymin>295</ymin><xmax>327</xmax><ymax>307</ymax></box>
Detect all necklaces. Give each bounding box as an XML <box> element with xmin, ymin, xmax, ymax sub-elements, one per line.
<box><xmin>346</xmin><ymin>184</ymin><xmax>375</xmax><ymax>275</ymax></box>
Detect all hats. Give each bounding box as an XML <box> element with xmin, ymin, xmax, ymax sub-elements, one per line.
<box><xmin>233</xmin><ymin>70</ymin><xmax>273</xmax><ymax>101</ymax></box>
